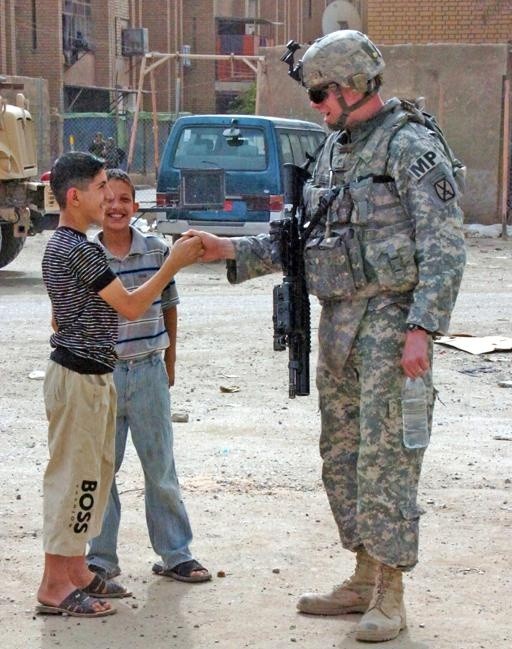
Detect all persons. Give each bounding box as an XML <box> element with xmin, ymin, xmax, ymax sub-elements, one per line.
<box><xmin>86</xmin><ymin>168</ymin><xmax>214</xmax><ymax>584</ymax></box>
<box><xmin>36</xmin><ymin>152</ymin><xmax>204</xmax><ymax>617</ymax></box>
<box><xmin>89</xmin><ymin>132</ymin><xmax>103</xmax><ymax>158</ymax></box>
<box><xmin>114</xmin><ymin>147</ymin><xmax>127</xmax><ymax>171</ymax></box>
<box><xmin>181</xmin><ymin>29</ymin><xmax>463</xmax><ymax>643</ymax></box>
<box><xmin>106</xmin><ymin>138</ymin><xmax>117</xmax><ymax>169</ymax></box>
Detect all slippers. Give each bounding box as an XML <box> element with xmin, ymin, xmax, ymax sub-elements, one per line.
<box><xmin>35</xmin><ymin>565</ymin><xmax>132</xmax><ymax>618</ymax></box>
<box><xmin>153</xmin><ymin>559</ymin><xmax>211</xmax><ymax>582</ymax></box>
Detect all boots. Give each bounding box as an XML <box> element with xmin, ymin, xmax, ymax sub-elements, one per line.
<box><xmin>355</xmin><ymin>564</ymin><xmax>407</xmax><ymax>642</ymax></box>
<box><xmin>296</xmin><ymin>556</ymin><xmax>379</xmax><ymax>615</ymax></box>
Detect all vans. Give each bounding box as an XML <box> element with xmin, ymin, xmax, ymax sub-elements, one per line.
<box><xmin>155</xmin><ymin>113</ymin><xmax>332</xmax><ymax>247</ymax></box>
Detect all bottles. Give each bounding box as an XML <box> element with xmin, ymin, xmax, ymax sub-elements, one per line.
<box><xmin>399</xmin><ymin>374</ymin><xmax>431</xmax><ymax>450</ymax></box>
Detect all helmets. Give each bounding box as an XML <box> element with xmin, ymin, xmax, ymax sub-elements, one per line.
<box><xmin>299</xmin><ymin>29</ymin><xmax>386</xmax><ymax>90</ymax></box>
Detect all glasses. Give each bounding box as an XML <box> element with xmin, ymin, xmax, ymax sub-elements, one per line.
<box><xmin>305</xmin><ymin>88</ymin><xmax>329</xmax><ymax>103</ymax></box>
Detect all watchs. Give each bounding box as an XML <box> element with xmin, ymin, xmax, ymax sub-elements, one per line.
<box><xmin>407</xmin><ymin>323</ymin><xmax>428</xmax><ymax>332</ymax></box>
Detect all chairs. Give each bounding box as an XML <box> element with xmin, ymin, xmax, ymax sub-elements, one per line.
<box><xmin>191</xmin><ymin>142</ymin><xmax>260</xmax><ymax>156</ymax></box>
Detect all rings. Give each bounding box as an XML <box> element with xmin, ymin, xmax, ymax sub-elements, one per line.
<box><xmin>416</xmin><ymin>369</ymin><xmax>424</xmax><ymax>373</ymax></box>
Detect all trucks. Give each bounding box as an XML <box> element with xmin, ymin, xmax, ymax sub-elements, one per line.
<box><xmin>0</xmin><ymin>91</ymin><xmax>62</xmax><ymax>268</ymax></box>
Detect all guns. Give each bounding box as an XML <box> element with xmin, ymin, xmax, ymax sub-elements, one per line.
<box><xmin>271</xmin><ymin>161</ymin><xmax>310</xmax><ymax>399</ymax></box>
